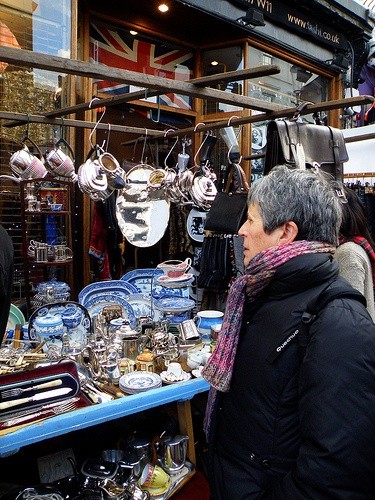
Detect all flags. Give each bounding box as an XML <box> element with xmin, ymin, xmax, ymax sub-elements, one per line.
<box><xmin>89</xmin><ymin>20</ymin><xmax>195</xmax><ymax>128</ymax></box>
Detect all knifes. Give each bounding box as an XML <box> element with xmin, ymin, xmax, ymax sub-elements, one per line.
<box><xmin>0</xmin><ymin>397</ymin><xmax>81</xmax><ymax>423</ymax></box>
<box><xmin>0</xmin><ymin>388</ymin><xmax>72</xmax><ymax>410</ymax></box>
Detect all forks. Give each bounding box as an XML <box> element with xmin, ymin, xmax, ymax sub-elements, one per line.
<box><xmin>2</xmin><ymin>401</ymin><xmax>78</xmax><ymax>427</ymax></box>
<box><xmin>1</xmin><ymin>378</ymin><xmax>63</xmax><ymax>399</ymax></box>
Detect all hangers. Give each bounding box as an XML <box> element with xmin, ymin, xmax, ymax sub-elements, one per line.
<box><xmin>344</xmin><ymin>172</ymin><xmax>375</xmax><ymax>192</ymax></box>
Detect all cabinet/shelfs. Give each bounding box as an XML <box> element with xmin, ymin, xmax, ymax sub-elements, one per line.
<box><xmin>0</xmin><ymin>330</ymin><xmax>236</xmax><ymax>500</ymax></box>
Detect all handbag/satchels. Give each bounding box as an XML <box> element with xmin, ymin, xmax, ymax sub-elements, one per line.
<box><xmin>263</xmin><ymin>101</ymin><xmax>349</xmax><ymax>186</ymax></box>
<box><xmin>203</xmin><ymin>164</ymin><xmax>252</xmax><ymax>236</ymax></box>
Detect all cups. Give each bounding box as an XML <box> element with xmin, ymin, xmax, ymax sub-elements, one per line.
<box><xmin>165</xmin><ymin>363</ymin><xmax>184</xmax><ymax>381</ymax></box>
<box><xmin>28</xmin><ymin>201</ymin><xmax>41</xmax><ymax>212</ymax></box>
<box><xmin>35</xmin><ymin>247</ymin><xmax>48</xmax><ymax>262</ymax></box>
<box><xmin>54</xmin><ymin>245</ymin><xmax>73</xmax><ymax>262</ymax></box>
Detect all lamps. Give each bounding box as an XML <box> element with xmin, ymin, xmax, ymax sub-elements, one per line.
<box><xmin>324</xmin><ymin>52</ymin><xmax>349</xmax><ymax>73</ymax></box>
<box><xmin>236</xmin><ymin>9</ymin><xmax>266</xmax><ymax>31</ymax></box>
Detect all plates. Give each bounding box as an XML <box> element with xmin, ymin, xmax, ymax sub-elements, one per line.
<box><xmin>119</xmin><ymin>371</ymin><xmax>163</xmax><ymax>394</ymax></box>
<box><xmin>160</xmin><ymin>370</ymin><xmax>191</xmax><ymax>384</ymax></box>
<box><xmin>78</xmin><ymin>269</ymin><xmax>195</xmax><ymax>330</ymax></box>
<box><xmin>7</xmin><ymin>304</ymin><xmax>25</xmax><ymax>329</ymax></box>
<box><xmin>252</xmin><ymin>127</ymin><xmax>267</xmax><ymax>150</ymax></box>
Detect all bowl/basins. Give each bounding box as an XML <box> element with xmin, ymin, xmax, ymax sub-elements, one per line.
<box><xmin>51</xmin><ymin>204</ymin><xmax>62</xmax><ymax>211</ymax></box>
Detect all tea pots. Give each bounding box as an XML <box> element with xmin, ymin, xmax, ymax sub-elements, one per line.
<box><xmin>68</xmin><ymin>428</ymin><xmax>190</xmax><ymax>500</ymax></box>
<box><xmin>45</xmin><ymin>315</ymin><xmax>183</xmax><ymax>386</ymax></box>
<box><xmin>141</xmin><ymin>167</ymin><xmax>217</xmax><ymax>212</ymax></box>
<box><xmin>176</xmin><ymin>316</ymin><xmax>201</xmax><ymax>340</ymax></box>
<box><xmin>78</xmin><ymin>144</ymin><xmax>119</xmax><ymax>203</ymax></box>
<box><xmin>0</xmin><ymin>137</ymin><xmax>48</xmax><ymax>184</ymax></box>
<box><xmin>44</xmin><ymin>138</ymin><xmax>78</xmax><ymax>182</ymax></box>
<box><xmin>157</xmin><ymin>258</ymin><xmax>192</xmax><ymax>279</ymax></box>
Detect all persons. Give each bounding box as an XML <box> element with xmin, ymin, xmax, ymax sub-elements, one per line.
<box><xmin>332</xmin><ymin>185</ymin><xmax>375</xmax><ymax>326</ymax></box>
<box><xmin>204</xmin><ymin>165</ymin><xmax>374</xmax><ymax>500</ymax></box>
